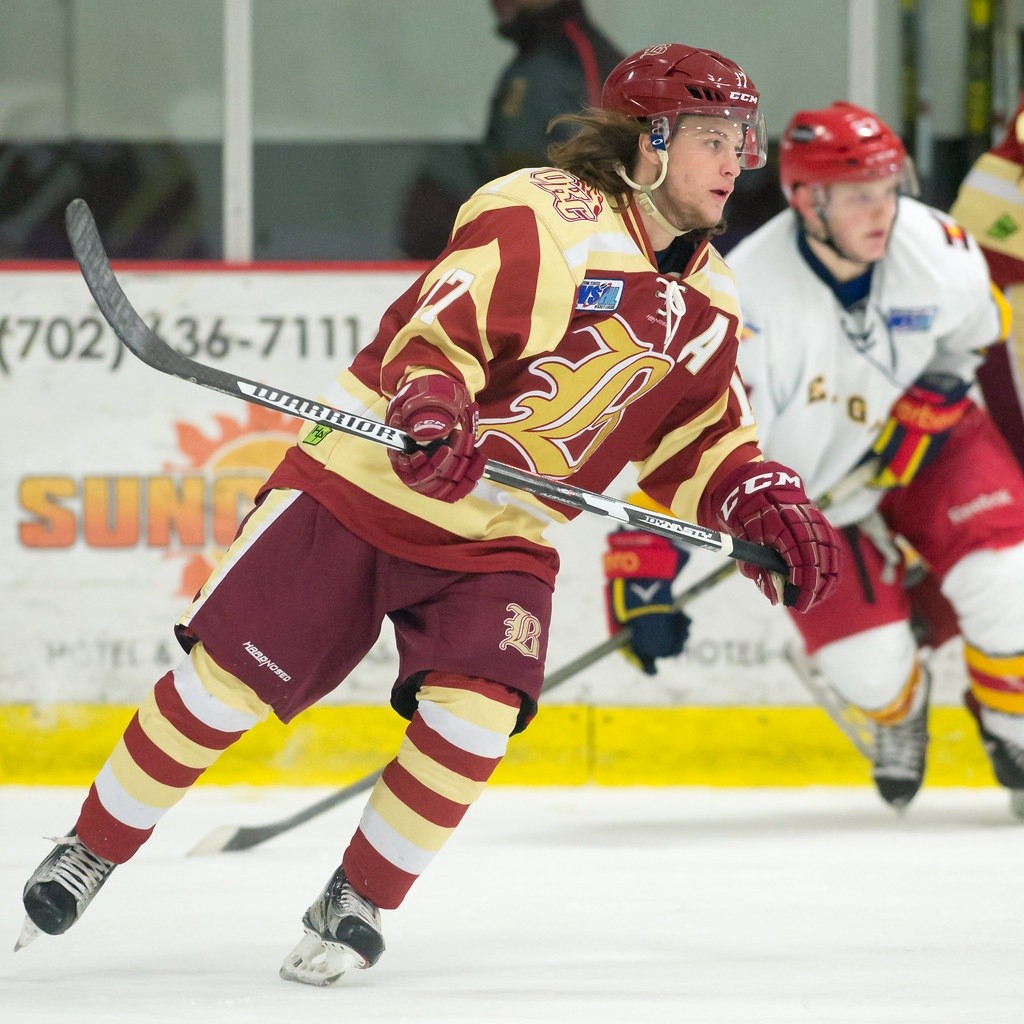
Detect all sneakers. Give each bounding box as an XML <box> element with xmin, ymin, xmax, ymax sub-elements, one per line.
<box><xmin>279</xmin><ymin>863</ymin><xmax>385</xmax><ymax>987</ymax></box>
<box><xmin>13</xmin><ymin>827</ymin><xmax>117</xmax><ymax>952</ymax></box>
<box><xmin>872</xmin><ymin>666</ymin><xmax>931</xmax><ymax>805</ymax></box>
<box><xmin>964</xmin><ymin>689</ymin><xmax>1024</xmax><ymax>819</ymax></box>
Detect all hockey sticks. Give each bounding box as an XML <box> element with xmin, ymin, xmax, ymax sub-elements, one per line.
<box><xmin>65</xmin><ymin>196</ymin><xmax>792</xmax><ymax>575</ymax></box>
<box><xmin>190</xmin><ymin>457</ymin><xmax>876</xmax><ymax>854</ymax></box>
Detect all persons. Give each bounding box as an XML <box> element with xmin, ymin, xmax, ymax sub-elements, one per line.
<box><xmin>600</xmin><ymin>100</ymin><xmax>1024</xmax><ymax>826</ymax></box>
<box><xmin>14</xmin><ymin>43</ymin><xmax>839</xmax><ymax>988</ymax></box>
<box><xmin>397</xmin><ymin>1</ymin><xmax>627</xmax><ymax>260</ymax></box>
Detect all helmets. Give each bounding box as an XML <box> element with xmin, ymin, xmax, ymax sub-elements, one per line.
<box><xmin>779</xmin><ymin>100</ymin><xmax>908</xmax><ymax>208</ymax></box>
<box><xmin>602</xmin><ymin>43</ymin><xmax>759</xmax><ymax>169</ymax></box>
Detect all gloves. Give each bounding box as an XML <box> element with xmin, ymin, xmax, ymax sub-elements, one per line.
<box><xmin>711</xmin><ymin>459</ymin><xmax>843</xmax><ymax>615</ymax></box>
<box><xmin>864</xmin><ymin>371</ymin><xmax>973</xmax><ymax>488</ymax></box>
<box><xmin>384</xmin><ymin>369</ymin><xmax>488</xmax><ymax>503</ymax></box>
<box><xmin>601</xmin><ymin>531</ymin><xmax>691</xmax><ymax>677</ymax></box>
